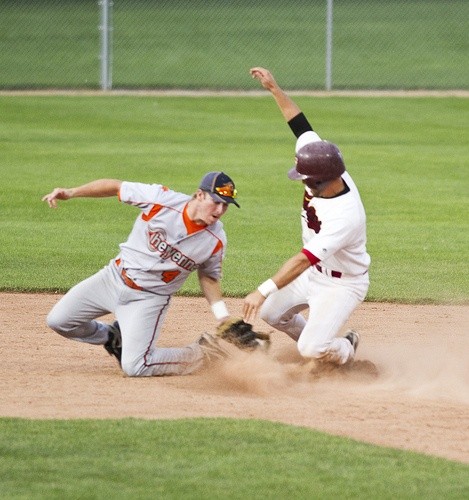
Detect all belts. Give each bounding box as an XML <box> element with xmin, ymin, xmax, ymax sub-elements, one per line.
<box><xmin>115</xmin><ymin>259</ymin><xmax>143</xmax><ymax>290</ymax></box>
<box><xmin>315</xmin><ymin>264</ymin><xmax>367</xmax><ymax>278</ymax></box>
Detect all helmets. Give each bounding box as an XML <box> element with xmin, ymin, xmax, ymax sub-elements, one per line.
<box><xmin>288</xmin><ymin>141</ymin><xmax>346</xmax><ymax>189</ymax></box>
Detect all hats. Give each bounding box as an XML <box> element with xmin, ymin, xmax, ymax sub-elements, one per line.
<box><xmin>200</xmin><ymin>171</ymin><xmax>240</xmax><ymax>208</ymax></box>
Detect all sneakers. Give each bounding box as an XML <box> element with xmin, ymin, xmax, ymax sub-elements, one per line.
<box><xmin>104</xmin><ymin>321</ymin><xmax>122</xmax><ymax>365</ymax></box>
<box><xmin>309</xmin><ymin>358</ymin><xmax>325</xmax><ymax>373</ymax></box>
<box><xmin>336</xmin><ymin>330</ymin><xmax>360</xmax><ymax>372</ymax></box>
<box><xmin>198</xmin><ymin>332</ymin><xmax>232</xmax><ymax>363</ymax></box>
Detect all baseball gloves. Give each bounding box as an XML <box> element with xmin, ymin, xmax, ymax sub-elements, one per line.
<box><xmin>216</xmin><ymin>316</ymin><xmax>270</xmax><ymax>355</ymax></box>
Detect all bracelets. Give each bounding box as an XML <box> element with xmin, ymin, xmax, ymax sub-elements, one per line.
<box><xmin>212</xmin><ymin>301</ymin><xmax>229</xmax><ymax>319</ymax></box>
<box><xmin>258</xmin><ymin>278</ymin><xmax>279</xmax><ymax>297</ymax></box>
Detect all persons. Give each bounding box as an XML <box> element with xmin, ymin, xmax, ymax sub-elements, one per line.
<box><xmin>42</xmin><ymin>171</ymin><xmax>260</xmax><ymax>376</ymax></box>
<box><xmin>242</xmin><ymin>66</ymin><xmax>371</xmax><ymax>368</ymax></box>
